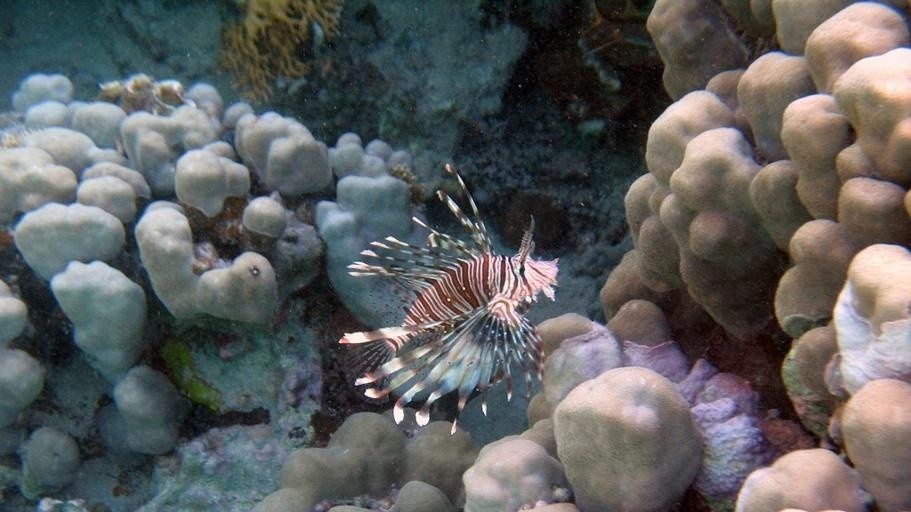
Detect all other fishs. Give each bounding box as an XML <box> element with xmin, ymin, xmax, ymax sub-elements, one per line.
<box><xmin>333</xmin><ymin>158</ymin><xmax>564</xmax><ymax>439</ymax></box>
